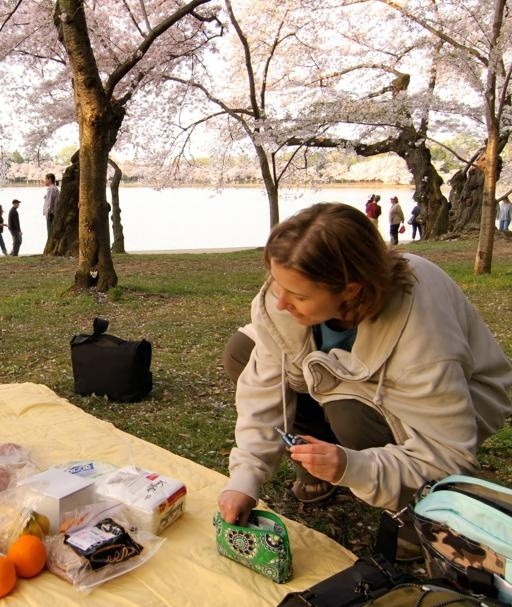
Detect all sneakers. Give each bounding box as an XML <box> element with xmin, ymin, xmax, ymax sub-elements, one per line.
<box><xmin>369</xmin><ymin>525</ymin><xmax>422</xmax><ymax>561</ymax></box>
<box><xmin>293</xmin><ymin>476</ymin><xmax>335</xmax><ymax>502</ymax></box>
<box><xmin>368</xmin><ymin>193</ymin><xmax>398</xmax><ymax>201</ymax></box>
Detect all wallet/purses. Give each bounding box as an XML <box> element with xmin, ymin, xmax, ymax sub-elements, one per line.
<box><xmin>215</xmin><ymin>508</ymin><xmax>297</xmax><ymax>583</ymax></box>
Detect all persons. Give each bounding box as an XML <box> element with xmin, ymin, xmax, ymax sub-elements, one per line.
<box><xmin>42</xmin><ymin>173</ymin><xmax>60</xmax><ymax>236</ymax></box>
<box><xmin>388</xmin><ymin>196</ymin><xmax>405</xmax><ymax>244</ymax></box>
<box><xmin>410</xmin><ymin>202</ymin><xmax>423</xmax><ymax>241</ymax></box>
<box><xmin>366</xmin><ymin>194</ymin><xmax>383</xmax><ymax>229</ymax></box>
<box><xmin>0</xmin><ymin>205</ymin><xmax>10</xmax><ymax>256</ymax></box>
<box><xmin>7</xmin><ymin>199</ymin><xmax>22</xmax><ymax>256</ymax></box>
<box><xmin>216</xmin><ymin>202</ymin><xmax>512</xmax><ymax>563</ymax></box>
<box><xmin>499</xmin><ymin>196</ymin><xmax>512</xmax><ymax>230</ymax></box>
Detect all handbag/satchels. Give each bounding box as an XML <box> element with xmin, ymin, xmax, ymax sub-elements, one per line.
<box><xmin>399</xmin><ymin>215</ymin><xmax>414</xmax><ymax>234</ymax></box>
<box><xmin>414</xmin><ymin>472</ymin><xmax>511</xmax><ymax>603</ymax></box>
<box><xmin>64</xmin><ymin>333</ymin><xmax>156</xmax><ymax>402</ymax></box>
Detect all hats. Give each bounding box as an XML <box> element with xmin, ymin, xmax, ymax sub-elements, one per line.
<box><xmin>11</xmin><ymin>199</ymin><xmax>22</xmax><ymax>205</ymax></box>
